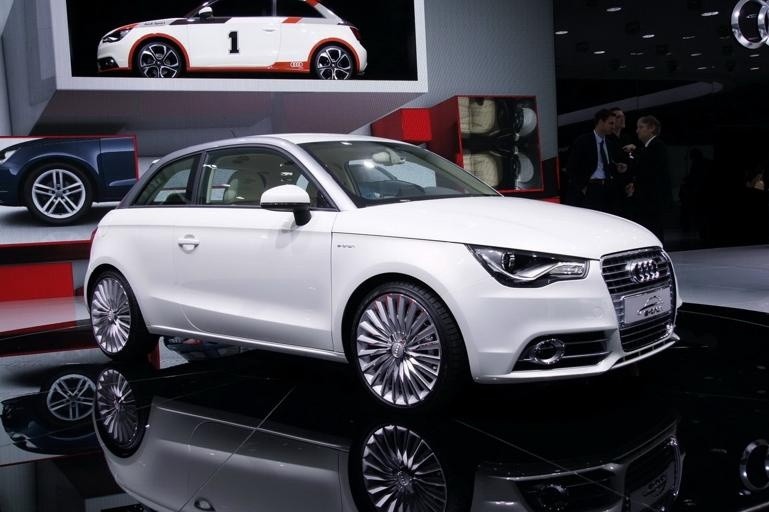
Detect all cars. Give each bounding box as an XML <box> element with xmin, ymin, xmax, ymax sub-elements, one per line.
<box><xmin>2</xmin><ymin>371</ymin><xmax>100</xmax><ymax>458</ymax></box>
<box><xmin>89</xmin><ymin>359</ymin><xmax>686</xmax><ymax>510</ymax></box>
<box><xmin>83</xmin><ymin>131</ymin><xmax>685</xmax><ymax>412</ymax></box>
<box><xmin>0</xmin><ymin>135</ymin><xmax>135</xmax><ymax>224</ymax></box>
<box><xmin>162</xmin><ymin>333</ymin><xmax>260</xmax><ymax>361</ymax></box>
<box><xmin>97</xmin><ymin>1</ymin><xmax>369</xmax><ymax>80</ymax></box>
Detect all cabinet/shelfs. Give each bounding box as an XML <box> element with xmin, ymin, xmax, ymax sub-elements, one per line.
<box><xmin>430</xmin><ymin>92</ymin><xmax>547</xmax><ymax>195</ymax></box>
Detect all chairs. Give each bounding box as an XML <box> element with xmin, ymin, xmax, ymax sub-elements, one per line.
<box><xmin>222</xmin><ymin>168</ymin><xmax>275</xmax><ymax>204</ymax></box>
<box><xmin>306</xmin><ymin>162</ymin><xmax>354</xmax><ymax>208</ymax></box>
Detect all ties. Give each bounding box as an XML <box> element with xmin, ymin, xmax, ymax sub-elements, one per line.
<box><xmin>598</xmin><ymin>140</ymin><xmax>612</xmax><ymax>178</ymax></box>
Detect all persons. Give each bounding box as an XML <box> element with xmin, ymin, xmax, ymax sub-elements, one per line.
<box><xmin>621</xmin><ymin>114</ymin><xmax>673</xmax><ymax>233</ymax></box>
<box><xmin>610</xmin><ymin>107</ymin><xmax>629</xmax><ymax>146</ymax></box>
<box><xmin>569</xmin><ymin>108</ymin><xmax>632</xmax><ymax>216</ymax></box>
